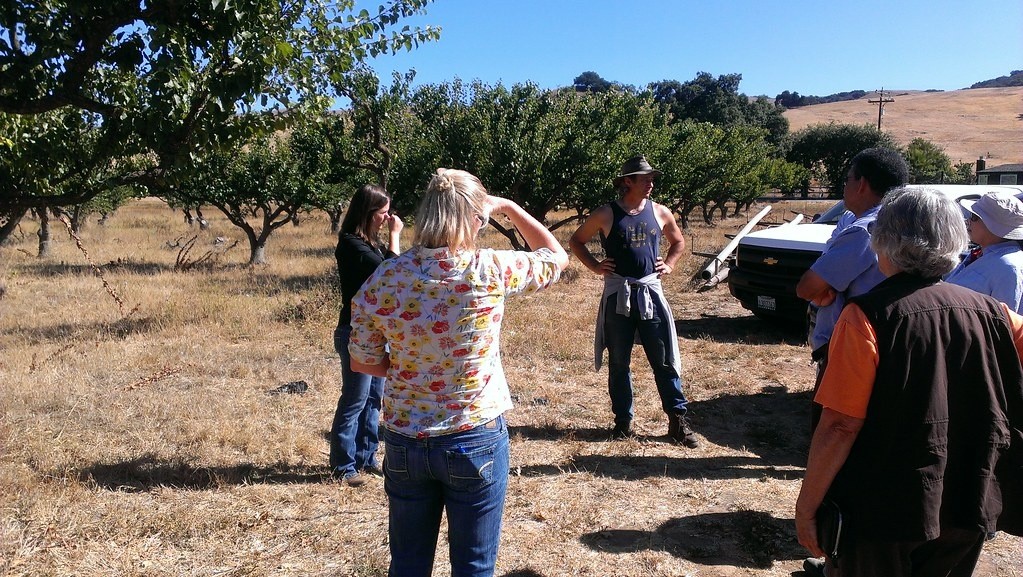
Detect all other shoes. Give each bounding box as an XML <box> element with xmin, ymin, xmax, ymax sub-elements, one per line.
<box><xmin>668</xmin><ymin>415</ymin><xmax>698</xmax><ymax>449</ymax></box>
<box><xmin>612</xmin><ymin>425</ymin><xmax>638</xmax><ymax>440</ymax></box>
<box><xmin>803</xmin><ymin>557</ymin><xmax>827</xmax><ymax>576</ymax></box>
<box><xmin>346</xmin><ymin>476</ymin><xmax>368</xmax><ymax>486</ymax></box>
<box><xmin>361</xmin><ymin>465</ymin><xmax>383</xmax><ymax>477</ymax></box>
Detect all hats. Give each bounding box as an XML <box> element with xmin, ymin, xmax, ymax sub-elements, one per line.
<box><xmin>960</xmin><ymin>191</ymin><xmax>1023</xmax><ymax>240</ymax></box>
<box><xmin>613</xmin><ymin>157</ymin><xmax>663</xmax><ymax>188</ymax></box>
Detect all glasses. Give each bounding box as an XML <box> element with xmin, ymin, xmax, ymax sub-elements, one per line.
<box><xmin>970</xmin><ymin>215</ymin><xmax>979</xmax><ymax>222</ymax></box>
<box><xmin>843</xmin><ymin>176</ymin><xmax>856</xmax><ymax>186</ymax></box>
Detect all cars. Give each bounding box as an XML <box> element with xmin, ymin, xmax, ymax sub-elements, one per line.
<box><xmin>727</xmin><ymin>182</ymin><xmax>1023</xmax><ymax>328</ymax></box>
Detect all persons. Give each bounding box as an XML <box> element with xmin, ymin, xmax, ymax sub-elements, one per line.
<box><xmin>796</xmin><ymin>148</ymin><xmax>909</xmax><ymax>434</ymax></box>
<box><xmin>346</xmin><ymin>169</ymin><xmax>569</xmax><ymax>577</ymax></box>
<box><xmin>794</xmin><ymin>187</ymin><xmax>1023</xmax><ymax>577</ymax></box>
<box><xmin>946</xmin><ymin>191</ymin><xmax>1023</xmax><ymax>316</ymax></box>
<box><xmin>330</xmin><ymin>184</ymin><xmax>404</xmax><ymax>485</ymax></box>
<box><xmin>569</xmin><ymin>157</ymin><xmax>698</xmax><ymax>448</ymax></box>
